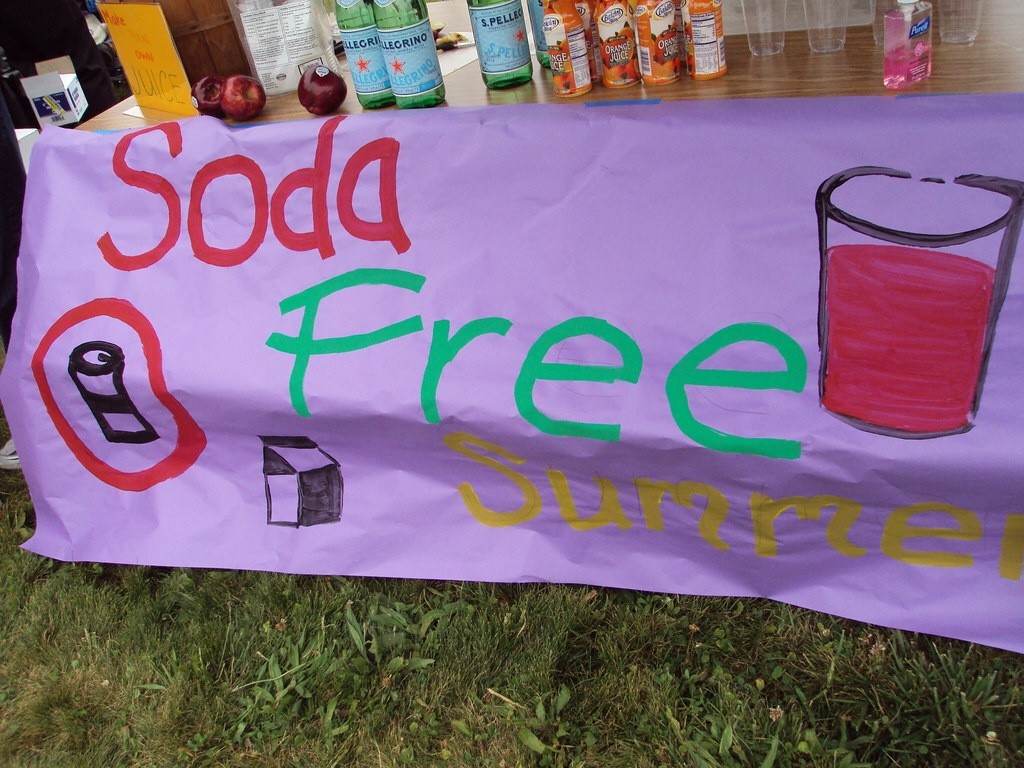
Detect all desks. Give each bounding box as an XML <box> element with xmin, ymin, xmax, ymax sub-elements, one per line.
<box><xmin>72</xmin><ymin>0</ymin><xmax>1024</xmax><ymax>132</ymax></box>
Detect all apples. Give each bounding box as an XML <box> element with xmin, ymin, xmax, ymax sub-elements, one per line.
<box><xmin>191</xmin><ymin>74</ymin><xmax>224</xmax><ymax>115</ymax></box>
<box><xmin>220</xmin><ymin>74</ymin><xmax>266</xmax><ymax>121</ymax></box>
<box><xmin>298</xmin><ymin>65</ymin><xmax>347</xmax><ymax>115</ymax></box>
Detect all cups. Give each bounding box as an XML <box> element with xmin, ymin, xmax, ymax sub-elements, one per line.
<box><xmin>741</xmin><ymin>0</ymin><xmax>787</xmax><ymax>56</ymax></box>
<box><xmin>803</xmin><ymin>0</ymin><xmax>849</xmax><ymax>53</ymax></box>
<box><xmin>936</xmin><ymin>0</ymin><xmax>982</xmax><ymax>44</ymax></box>
<box><xmin>869</xmin><ymin>0</ymin><xmax>898</xmax><ymax>46</ymax></box>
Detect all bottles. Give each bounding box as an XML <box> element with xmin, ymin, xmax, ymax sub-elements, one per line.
<box><xmin>466</xmin><ymin>0</ymin><xmax>728</xmax><ymax>97</ymax></box>
<box><xmin>334</xmin><ymin>0</ymin><xmax>396</xmax><ymax>109</ymax></box>
<box><xmin>884</xmin><ymin>0</ymin><xmax>932</xmax><ymax>90</ymax></box>
<box><xmin>373</xmin><ymin>0</ymin><xmax>445</xmax><ymax>109</ymax></box>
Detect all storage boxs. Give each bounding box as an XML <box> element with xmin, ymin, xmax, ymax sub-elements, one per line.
<box><xmin>20</xmin><ymin>55</ymin><xmax>88</xmax><ymax>129</ymax></box>
